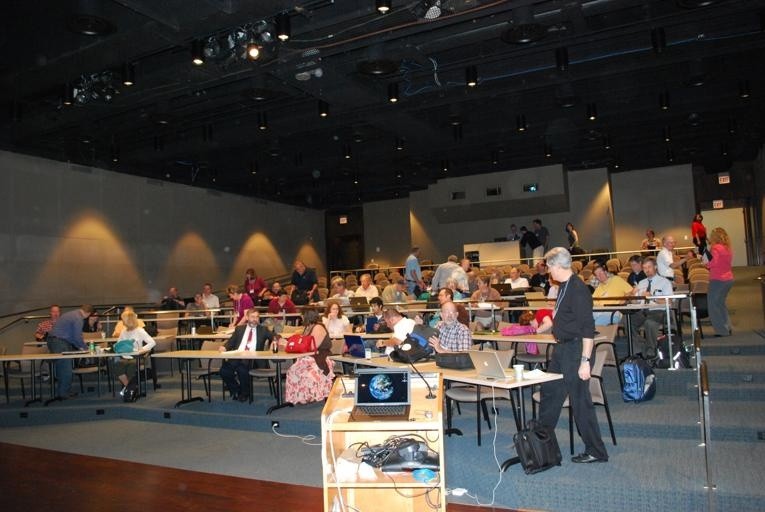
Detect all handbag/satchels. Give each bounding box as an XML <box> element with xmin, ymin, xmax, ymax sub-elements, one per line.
<box><xmin>500</xmin><ymin>324</ymin><xmax>539</xmax><ymax>354</ymax></box>
<box><xmin>513</xmin><ymin>420</ymin><xmax>562</xmax><ymax>474</ymax></box>
<box><xmin>285</xmin><ymin>335</ymin><xmax>317</xmax><ymax>354</ymax></box>
<box><xmin>113</xmin><ymin>339</ymin><xmax>134</xmax><ymax>354</ymax></box>
<box><xmin>391</xmin><ymin>324</ymin><xmax>439</xmax><ymax>365</ymax></box>
<box><xmin>619</xmin><ymin>355</ymin><xmax>655</xmax><ymax>402</ymax></box>
<box><xmin>656</xmin><ymin>333</ymin><xmax>689</xmax><ymax>368</ymax></box>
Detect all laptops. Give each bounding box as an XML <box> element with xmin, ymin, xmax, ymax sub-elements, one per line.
<box><xmin>344</xmin><ymin>335</ymin><xmax>388</xmax><ymax>359</ymax></box>
<box><xmin>491</xmin><ymin>284</ymin><xmax>511</xmax><ymax>296</ymax></box>
<box><xmin>63</xmin><ymin>351</ymin><xmax>89</xmax><ymax>354</ymax></box>
<box><xmin>469</xmin><ymin>350</ymin><xmax>513</xmax><ymax>377</ymax></box>
<box><xmin>349</xmin><ymin>297</ymin><xmax>370</xmax><ymax>312</ymax></box>
<box><xmin>347</xmin><ymin>368</ymin><xmax>410</xmax><ymax>422</ymax></box>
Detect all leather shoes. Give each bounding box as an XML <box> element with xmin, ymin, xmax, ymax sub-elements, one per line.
<box><xmin>572</xmin><ymin>453</ymin><xmax>608</xmax><ymax>463</ymax></box>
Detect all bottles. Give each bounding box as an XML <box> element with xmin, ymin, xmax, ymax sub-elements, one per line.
<box><xmin>396</xmin><ymin>289</ymin><xmax>401</xmax><ymax>303</ymax></box>
<box><xmin>191</xmin><ymin>320</ymin><xmax>196</xmax><ymax>334</ymax></box>
<box><xmin>90</xmin><ymin>339</ymin><xmax>94</xmax><ymax>352</ymax></box>
<box><xmin>273</xmin><ymin>335</ymin><xmax>278</xmax><ymax>353</ymax></box>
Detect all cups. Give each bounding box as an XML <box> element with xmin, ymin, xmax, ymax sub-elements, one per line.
<box><xmin>513</xmin><ymin>365</ymin><xmax>525</xmax><ymax>379</ymax></box>
<box><xmin>365</xmin><ymin>348</ymin><xmax>372</xmax><ymax>360</ymax></box>
<box><xmin>101</xmin><ymin>333</ymin><xmax>106</xmax><ymax>338</ymax></box>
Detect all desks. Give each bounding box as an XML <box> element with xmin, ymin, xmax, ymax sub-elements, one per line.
<box><xmin>464</xmin><ymin>240</ymin><xmax>526</xmax><ymax>265</ymax></box>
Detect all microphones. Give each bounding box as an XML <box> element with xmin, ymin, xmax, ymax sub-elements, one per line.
<box><xmin>398</xmin><ymin>351</ymin><xmax>436</xmax><ymax>399</ymax></box>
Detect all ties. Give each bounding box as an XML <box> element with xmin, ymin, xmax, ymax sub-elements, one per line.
<box><xmin>245</xmin><ymin>329</ymin><xmax>252</xmax><ymax>351</ymax></box>
<box><xmin>645</xmin><ymin>280</ymin><xmax>653</xmax><ymax>303</ymax></box>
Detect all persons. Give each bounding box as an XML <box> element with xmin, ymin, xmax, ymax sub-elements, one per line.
<box><xmin>688</xmin><ymin>214</ymin><xmax>734</xmax><ymax>336</ymax></box>
<box><xmin>219</xmin><ymin>261</ymin><xmax>336</xmax><ymax>403</ymax></box>
<box><xmin>572</xmin><ymin>260</ymin><xmax>636</xmax><ymax>324</ymax></box>
<box><xmin>507</xmin><ymin>219</ymin><xmax>549</xmax><ymax>249</ymax></box>
<box><xmin>565</xmin><ymin>223</ymin><xmax>578</xmax><ymax>249</ymax></box>
<box><xmin>112</xmin><ymin>304</ymin><xmax>156</xmax><ymax>395</ymax></box>
<box><xmin>539</xmin><ymin>247</ymin><xmax>608</xmax><ymax>464</ymax></box>
<box><xmin>320</xmin><ymin>248</ymin><xmax>558</xmax><ymax>354</ymax></box>
<box><xmin>35</xmin><ymin>303</ymin><xmax>103</xmax><ymax>401</ymax></box>
<box><xmin>628</xmin><ymin>229</ymin><xmax>686</xmax><ymax>358</ymax></box>
<box><xmin>163</xmin><ymin>284</ymin><xmax>219</xmax><ymax>327</ymax></box>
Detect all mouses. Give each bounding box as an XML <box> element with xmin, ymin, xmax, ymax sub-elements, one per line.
<box><xmin>425</xmin><ymin>410</ymin><xmax>433</xmax><ymax>419</ymax></box>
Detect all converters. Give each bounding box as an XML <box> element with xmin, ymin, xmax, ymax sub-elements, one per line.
<box><xmin>452</xmin><ymin>487</ymin><xmax>465</xmax><ymax>496</ymax></box>
